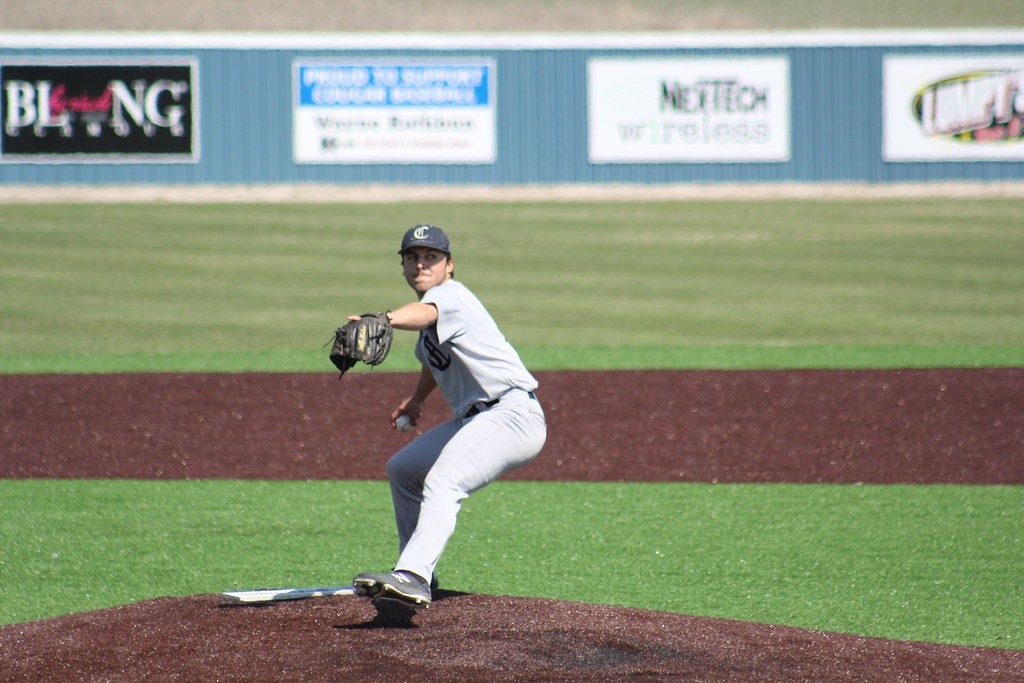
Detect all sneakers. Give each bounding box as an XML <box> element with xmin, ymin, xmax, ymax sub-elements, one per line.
<box><xmin>353</xmin><ymin>571</ymin><xmax>432</xmax><ymax>611</ymax></box>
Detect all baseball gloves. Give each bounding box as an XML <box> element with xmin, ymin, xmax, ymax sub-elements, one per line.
<box><xmin>329</xmin><ymin>312</ymin><xmax>393</xmax><ymax>381</ymax></box>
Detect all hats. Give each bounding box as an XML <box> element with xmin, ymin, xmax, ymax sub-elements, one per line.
<box><xmin>399</xmin><ymin>225</ymin><xmax>450</xmax><ymax>254</ymax></box>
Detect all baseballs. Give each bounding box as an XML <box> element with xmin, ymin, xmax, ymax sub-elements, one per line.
<box><xmin>394</xmin><ymin>414</ymin><xmax>412</xmax><ymax>432</ymax></box>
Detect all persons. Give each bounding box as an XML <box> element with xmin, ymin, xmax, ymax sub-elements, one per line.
<box><xmin>327</xmin><ymin>224</ymin><xmax>546</xmax><ymax>611</ymax></box>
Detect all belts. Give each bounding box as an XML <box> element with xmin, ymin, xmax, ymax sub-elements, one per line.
<box><xmin>462</xmin><ymin>392</ymin><xmax>538</xmax><ymax>418</ymax></box>
